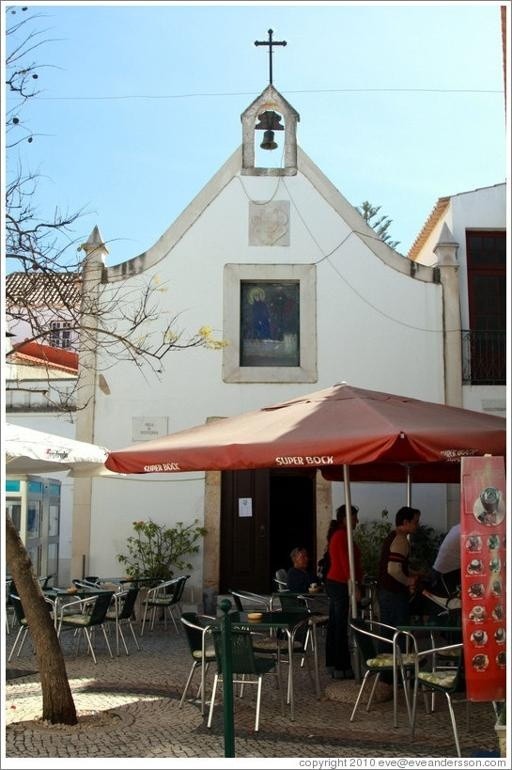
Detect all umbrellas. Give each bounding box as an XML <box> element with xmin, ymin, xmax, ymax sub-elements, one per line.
<box><xmin>105</xmin><ymin>382</ymin><xmax>506</xmax><ymax>679</ymax></box>
<box><xmin>6</xmin><ymin>423</ymin><xmax>107</xmax><ymax>479</ymax></box>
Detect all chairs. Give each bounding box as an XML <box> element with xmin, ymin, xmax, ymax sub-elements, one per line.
<box><xmin>36</xmin><ymin>576</ymin><xmax>58</xmax><ymax>592</ymax></box>
<box><xmin>409</xmin><ymin>641</ymin><xmax>506</xmax><ymax>757</ymax></box>
<box><xmin>140</xmin><ymin>575</ymin><xmax>191</xmax><ymax>636</ymax></box>
<box><xmin>8</xmin><ymin>594</ymin><xmax>57</xmax><ymax>661</ymax></box>
<box><xmin>86</xmin><ymin>588</ymin><xmax>141</xmax><ymax>655</ymax></box>
<box><xmin>72</xmin><ymin>578</ymin><xmax>109</xmax><ymax>638</ymax></box>
<box><xmin>229</xmin><ymin>588</ymin><xmax>273</xmax><ymax>613</ymax></box>
<box><xmin>207</xmin><ymin>628</ymin><xmax>287</xmax><ymax>732</ymax></box>
<box><xmin>178</xmin><ymin>610</ymin><xmax>239</xmax><ymax>709</ymax></box>
<box><xmin>270</xmin><ymin>592</ymin><xmax>312</xmax><ymax>667</ymax></box>
<box><xmin>56</xmin><ymin>591</ymin><xmax>115</xmax><ymax>664</ymax></box>
<box><xmin>344</xmin><ymin>616</ymin><xmax>415</xmax><ymax>729</ymax></box>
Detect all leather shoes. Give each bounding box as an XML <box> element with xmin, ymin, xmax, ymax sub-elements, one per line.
<box><xmin>334</xmin><ymin>673</ymin><xmax>355</xmax><ymax>680</ymax></box>
<box><xmin>328</xmin><ymin>671</ymin><xmax>333</xmax><ymax>673</ymax></box>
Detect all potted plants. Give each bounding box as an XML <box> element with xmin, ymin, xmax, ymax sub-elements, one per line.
<box><xmin>115</xmin><ymin>517</ymin><xmax>209</xmax><ymax>621</ymax></box>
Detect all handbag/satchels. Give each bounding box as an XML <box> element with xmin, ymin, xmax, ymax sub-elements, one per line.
<box><xmin>317</xmin><ymin>544</ymin><xmax>331</xmax><ymax>576</ymax></box>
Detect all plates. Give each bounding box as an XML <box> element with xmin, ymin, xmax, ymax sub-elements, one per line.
<box><xmin>248</xmin><ymin>613</ymin><xmax>262</xmax><ymax>619</ymax></box>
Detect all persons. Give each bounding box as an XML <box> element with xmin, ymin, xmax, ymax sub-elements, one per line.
<box><xmin>287</xmin><ymin>548</ymin><xmax>327</xmax><ymax>627</ymax></box>
<box><xmin>430</xmin><ymin>522</ymin><xmax>460</xmax><ymax>645</ymax></box>
<box><xmin>376</xmin><ymin>506</ymin><xmax>428</xmax><ymax>687</ymax></box>
<box><xmin>323</xmin><ymin>504</ymin><xmax>362</xmax><ymax>681</ymax></box>
